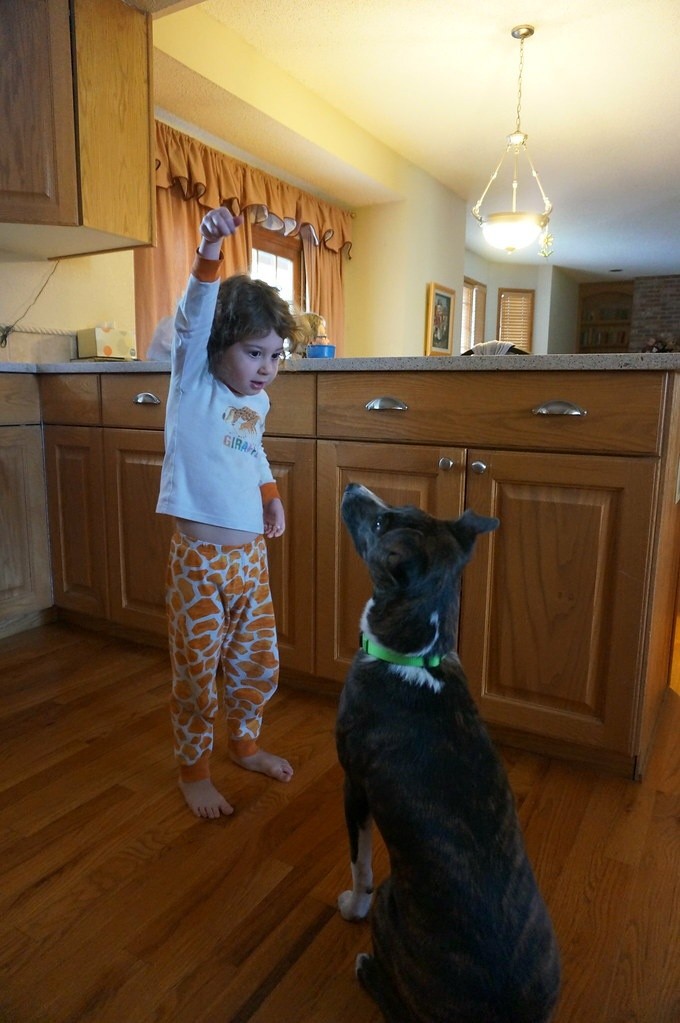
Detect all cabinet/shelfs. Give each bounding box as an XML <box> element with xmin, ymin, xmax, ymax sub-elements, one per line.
<box><xmin>574</xmin><ymin>281</ymin><xmax>633</xmax><ymax>353</ymax></box>
<box><xmin>0</xmin><ymin>374</ymin><xmax>680</xmax><ymax>784</ymax></box>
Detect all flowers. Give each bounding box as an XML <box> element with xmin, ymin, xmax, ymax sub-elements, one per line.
<box><xmin>637</xmin><ymin>331</ymin><xmax>677</xmax><ymax>353</ymax></box>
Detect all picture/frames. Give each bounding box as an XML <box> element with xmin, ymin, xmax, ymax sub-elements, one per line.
<box><xmin>426</xmin><ymin>282</ymin><xmax>455</xmax><ymax>356</ymax></box>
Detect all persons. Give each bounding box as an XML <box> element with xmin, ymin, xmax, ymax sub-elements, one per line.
<box><xmin>290</xmin><ymin>313</ymin><xmax>329</xmax><ymax>361</ymax></box>
<box><xmin>155</xmin><ymin>207</ymin><xmax>299</xmax><ymax>819</ymax></box>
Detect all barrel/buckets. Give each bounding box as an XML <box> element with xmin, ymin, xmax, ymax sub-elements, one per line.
<box><xmin>305</xmin><ymin>344</ymin><xmax>336</xmax><ymax>358</ymax></box>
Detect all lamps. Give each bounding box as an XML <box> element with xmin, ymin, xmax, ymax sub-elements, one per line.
<box><xmin>472</xmin><ymin>24</ymin><xmax>554</xmax><ymax>258</ymax></box>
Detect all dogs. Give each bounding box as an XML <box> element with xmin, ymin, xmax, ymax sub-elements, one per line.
<box><xmin>333</xmin><ymin>482</ymin><xmax>561</xmax><ymax>1023</ymax></box>
